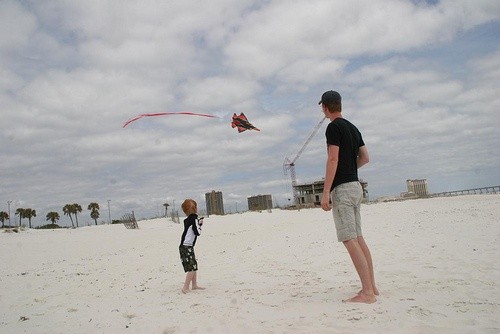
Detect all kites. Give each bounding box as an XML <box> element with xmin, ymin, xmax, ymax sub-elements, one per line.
<box><xmin>117</xmin><ymin>111</ymin><xmax>261</xmax><ymax>134</ymax></box>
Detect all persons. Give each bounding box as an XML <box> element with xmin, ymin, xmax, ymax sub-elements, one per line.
<box><xmin>317</xmin><ymin>90</ymin><xmax>379</xmax><ymax>304</ymax></box>
<box><xmin>178</xmin><ymin>198</ymin><xmax>206</xmax><ymax>294</ymax></box>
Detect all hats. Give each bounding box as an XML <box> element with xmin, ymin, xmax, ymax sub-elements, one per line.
<box><xmin>318</xmin><ymin>90</ymin><xmax>340</xmax><ymax>104</ymax></box>
